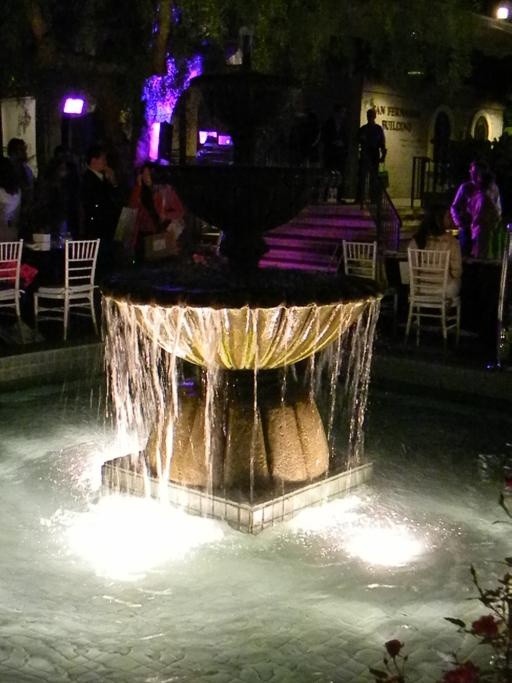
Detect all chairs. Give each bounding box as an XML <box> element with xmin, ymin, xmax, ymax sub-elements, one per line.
<box><xmin>404</xmin><ymin>248</ymin><xmax>461</xmax><ymax>348</ymax></box>
<box><xmin>33</xmin><ymin>238</ymin><xmax>101</xmax><ymax>340</ymax></box>
<box><xmin>342</xmin><ymin>239</ymin><xmax>398</xmax><ymax>320</ymax></box>
<box><xmin>0</xmin><ymin>239</ymin><xmax>25</xmax><ymax>345</ymax></box>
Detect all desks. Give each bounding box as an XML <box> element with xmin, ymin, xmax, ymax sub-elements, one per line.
<box><xmin>383</xmin><ymin>250</ymin><xmax>503</xmax><ymax>265</ymax></box>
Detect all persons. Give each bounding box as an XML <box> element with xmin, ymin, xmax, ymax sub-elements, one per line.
<box><xmin>7</xmin><ymin>137</ymin><xmax>40</xmax><ymax>193</ymax></box>
<box><xmin>288</xmin><ymin>100</ymin><xmax>320</xmax><ymax>163</ymax></box>
<box><xmin>125</xmin><ymin>161</ymin><xmax>190</xmax><ymax>255</ymax></box>
<box><xmin>66</xmin><ymin>147</ymin><xmax>126</xmax><ymax>270</ymax></box>
<box><xmin>1</xmin><ymin>157</ymin><xmax>29</xmax><ymax>252</ymax></box>
<box><xmin>451</xmin><ymin>161</ymin><xmax>502</xmax><ymax>255</ymax></box>
<box><xmin>403</xmin><ymin>202</ymin><xmax>469</xmax><ymax>305</ymax></box>
<box><xmin>352</xmin><ymin>106</ymin><xmax>387</xmax><ymax>205</ymax></box>
<box><xmin>468</xmin><ymin>171</ymin><xmax>498</xmax><ymax>258</ymax></box>
<box><xmin>320</xmin><ymin>101</ymin><xmax>353</xmax><ymax>196</ymax></box>
